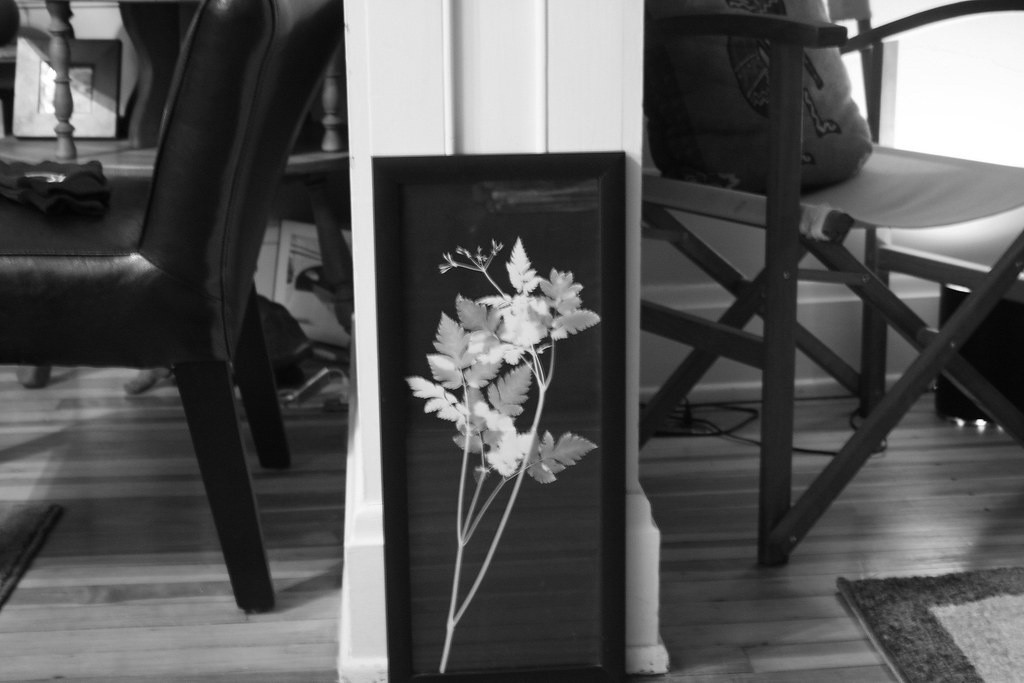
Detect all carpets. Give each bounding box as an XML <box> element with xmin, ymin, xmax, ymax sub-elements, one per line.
<box><xmin>0</xmin><ymin>501</ymin><xmax>65</xmax><ymax>608</ymax></box>
<box><xmin>838</xmin><ymin>568</ymin><xmax>1024</xmax><ymax>683</ymax></box>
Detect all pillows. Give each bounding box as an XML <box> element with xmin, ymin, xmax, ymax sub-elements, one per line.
<box><xmin>648</xmin><ymin>1</ymin><xmax>873</xmax><ymax>193</ymax></box>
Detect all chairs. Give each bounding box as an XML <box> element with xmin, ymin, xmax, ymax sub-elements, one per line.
<box><xmin>641</xmin><ymin>1</ymin><xmax>1024</xmax><ymax>573</ymax></box>
<box><xmin>0</xmin><ymin>0</ymin><xmax>343</xmax><ymax>616</ymax></box>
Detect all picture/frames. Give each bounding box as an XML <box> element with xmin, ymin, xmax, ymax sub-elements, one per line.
<box><xmin>370</xmin><ymin>152</ymin><xmax>628</xmax><ymax>682</ymax></box>
